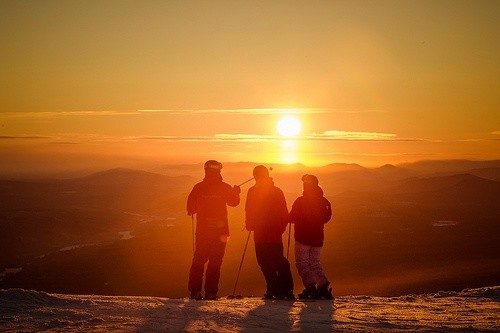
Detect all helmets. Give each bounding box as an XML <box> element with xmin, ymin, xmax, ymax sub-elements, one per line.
<box><xmin>204</xmin><ymin>160</ymin><xmax>222</xmax><ymax>170</ymax></box>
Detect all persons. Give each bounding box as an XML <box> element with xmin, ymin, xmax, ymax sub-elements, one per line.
<box><xmin>287</xmin><ymin>174</ymin><xmax>334</xmax><ymax>300</ymax></box>
<box><xmin>186</xmin><ymin>160</ymin><xmax>241</xmax><ymax>300</ymax></box>
<box><xmin>245</xmin><ymin>165</ymin><xmax>295</xmax><ymax>300</ymax></box>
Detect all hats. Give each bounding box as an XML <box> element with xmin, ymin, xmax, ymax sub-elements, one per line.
<box><xmin>302</xmin><ymin>174</ymin><xmax>319</xmax><ymax>186</ymax></box>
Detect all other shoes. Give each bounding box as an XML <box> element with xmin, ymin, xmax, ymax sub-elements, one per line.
<box><xmin>316</xmin><ymin>283</ymin><xmax>334</xmax><ymax>300</ymax></box>
<box><xmin>298</xmin><ymin>283</ymin><xmax>316</xmax><ymax>298</ymax></box>
<box><xmin>190</xmin><ymin>292</ymin><xmax>203</xmax><ymax>299</ymax></box>
<box><xmin>264</xmin><ymin>289</ymin><xmax>294</xmax><ymax>299</ymax></box>
<box><xmin>204</xmin><ymin>294</ymin><xmax>217</xmax><ymax>300</ymax></box>
